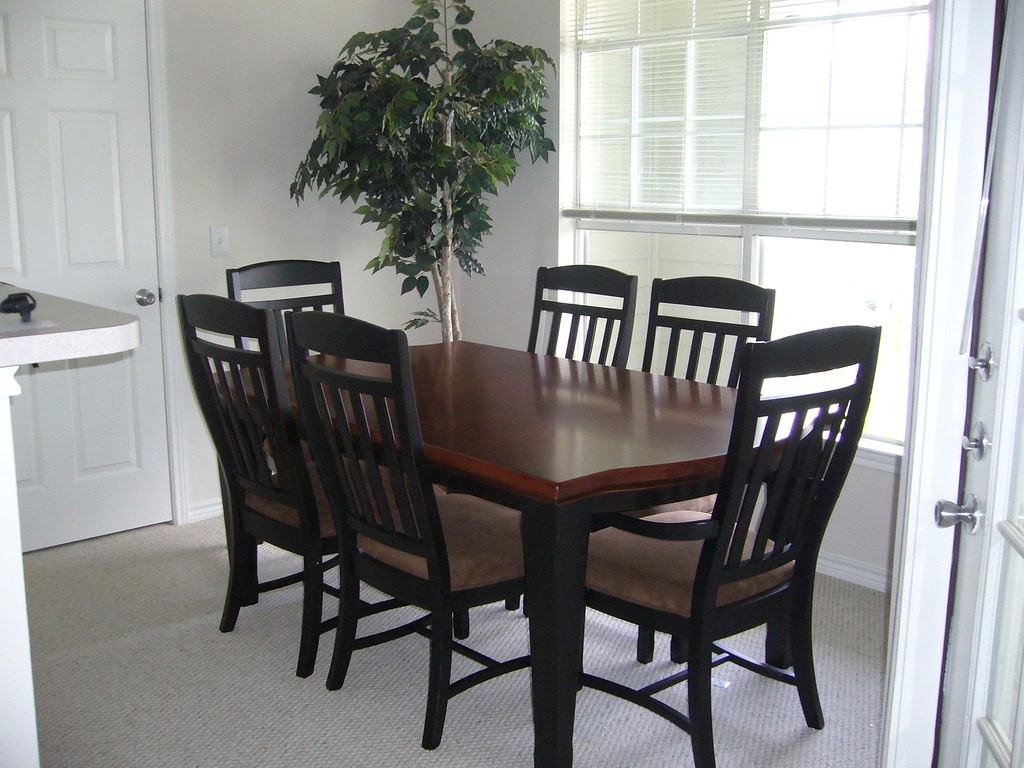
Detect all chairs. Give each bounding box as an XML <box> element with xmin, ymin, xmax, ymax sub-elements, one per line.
<box><xmin>505</xmin><ymin>264</ymin><xmax>638</xmax><ymax>612</ymax></box>
<box><xmin>583</xmin><ymin>324</ymin><xmax>882</xmax><ymax>768</ymax></box>
<box><xmin>284</xmin><ymin>309</ymin><xmax>532</xmax><ymax>749</ymax></box>
<box><xmin>176</xmin><ymin>294</ymin><xmax>472</xmax><ymax>678</ymax></box>
<box><xmin>225</xmin><ymin>259</ymin><xmax>345</xmax><ymax>368</ymax></box>
<box><xmin>523</xmin><ymin>275</ymin><xmax>775</xmax><ymax>667</ymax></box>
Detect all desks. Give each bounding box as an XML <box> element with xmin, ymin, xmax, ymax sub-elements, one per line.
<box><xmin>213</xmin><ymin>340</ymin><xmax>848</xmax><ymax>768</ymax></box>
<box><xmin>0</xmin><ymin>282</ymin><xmax>140</xmax><ymax>768</ymax></box>
